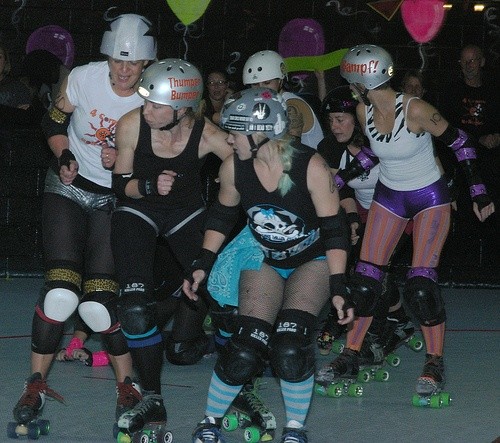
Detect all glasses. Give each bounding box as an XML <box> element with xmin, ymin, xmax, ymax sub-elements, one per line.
<box><xmin>457</xmin><ymin>58</ymin><xmax>483</xmax><ymax>67</ymax></box>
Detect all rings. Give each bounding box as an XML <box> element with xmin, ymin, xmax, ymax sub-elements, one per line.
<box><xmin>108</xmin><ymin>159</ymin><xmax>111</xmax><ymax>163</ymax></box>
<box><xmin>194</xmin><ymin>282</ymin><xmax>201</xmax><ymax>286</ymax></box>
<box><xmin>107</xmin><ymin>154</ymin><xmax>110</xmax><ymax>159</ymax></box>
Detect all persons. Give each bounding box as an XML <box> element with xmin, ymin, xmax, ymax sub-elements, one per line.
<box><xmin>317</xmin><ymin>88</ymin><xmax>426</xmax><ymax>381</ymax></box>
<box><xmin>242</xmin><ymin>49</ymin><xmax>354</xmax><ymax>357</ymax></box>
<box><xmin>108</xmin><ymin>59</ymin><xmax>238</xmax><ymax>443</ymax></box>
<box><xmin>200</xmin><ymin>68</ymin><xmax>238</xmax><ymax>207</ymax></box>
<box><xmin>58</xmin><ymin>310</ymin><xmax>217</xmax><ymax>366</ymax></box>
<box><xmin>313</xmin><ymin>55</ymin><xmax>328</xmax><ymax>109</ymax></box>
<box><xmin>441</xmin><ymin>45</ymin><xmax>500</xmax><ymax>287</ymax></box>
<box><xmin>311</xmin><ymin>45</ymin><xmax>498</xmax><ymax>410</ymax></box>
<box><xmin>0</xmin><ymin>45</ymin><xmax>32</xmax><ymax>267</ymax></box>
<box><xmin>7</xmin><ymin>13</ymin><xmax>162</xmax><ymax>443</ymax></box>
<box><xmin>181</xmin><ymin>89</ymin><xmax>356</xmax><ymax>443</ymax></box>
<box><xmin>401</xmin><ymin>70</ymin><xmax>429</xmax><ymax>102</ymax></box>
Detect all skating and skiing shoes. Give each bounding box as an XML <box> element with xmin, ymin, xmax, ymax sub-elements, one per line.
<box><xmin>7</xmin><ymin>308</ymin><xmax>451</xmax><ymax>443</ymax></box>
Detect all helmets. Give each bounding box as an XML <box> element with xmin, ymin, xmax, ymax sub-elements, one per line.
<box><xmin>340</xmin><ymin>44</ymin><xmax>395</xmax><ymax>89</ymax></box>
<box><xmin>242</xmin><ymin>50</ymin><xmax>289</xmax><ymax>85</ymax></box>
<box><xmin>101</xmin><ymin>13</ymin><xmax>158</xmax><ymax>59</ymax></box>
<box><xmin>319</xmin><ymin>86</ymin><xmax>361</xmax><ymax>113</ymax></box>
<box><xmin>136</xmin><ymin>58</ymin><xmax>203</xmax><ymax>112</ymax></box>
<box><xmin>220</xmin><ymin>87</ymin><xmax>289</xmax><ymax>140</ymax></box>
